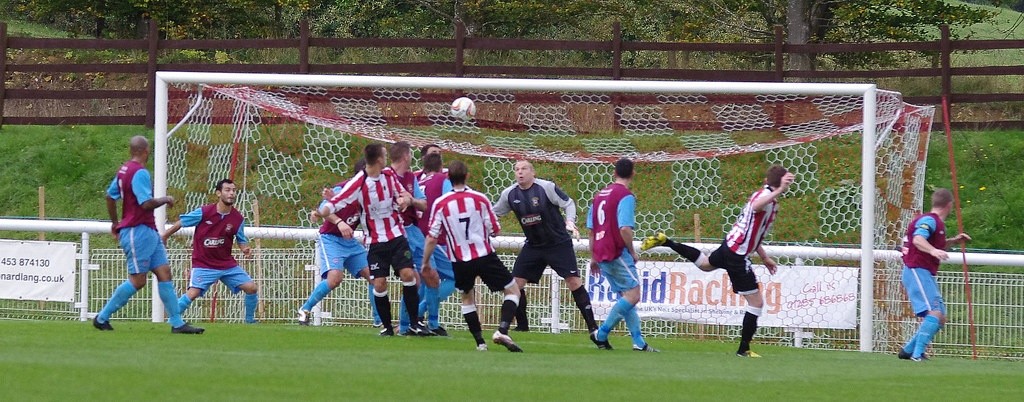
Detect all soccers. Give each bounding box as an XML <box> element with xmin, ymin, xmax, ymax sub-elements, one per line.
<box><xmin>451</xmin><ymin>97</ymin><xmax>475</xmax><ymax>122</ymax></box>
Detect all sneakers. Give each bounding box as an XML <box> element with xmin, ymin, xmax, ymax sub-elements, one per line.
<box><xmin>477</xmin><ymin>343</ymin><xmax>488</xmax><ymax>351</ymax></box>
<box><xmin>493</xmin><ymin>330</ymin><xmax>523</xmax><ymax>352</ymax></box>
<box><xmin>636</xmin><ymin>233</ymin><xmax>667</xmax><ymax>253</ymax></box>
<box><xmin>736</xmin><ymin>349</ymin><xmax>762</xmax><ymax>359</ymax></box>
<box><xmin>171</xmin><ymin>323</ymin><xmax>204</xmax><ymax>334</ymax></box>
<box><xmin>93</xmin><ymin>315</ymin><xmax>113</xmax><ymax>331</ymax></box>
<box><xmin>898</xmin><ymin>348</ymin><xmax>927</xmax><ymax>359</ymax></box>
<box><xmin>406</xmin><ymin>324</ymin><xmax>435</xmax><ymax>338</ymax></box>
<box><xmin>426</xmin><ymin>325</ymin><xmax>447</xmax><ymax>336</ymax></box>
<box><xmin>590</xmin><ymin>330</ymin><xmax>612</xmax><ymax>350</ymax></box>
<box><xmin>378</xmin><ymin>328</ymin><xmax>394</xmax><ymax>336</ymax></box>
<box><xmin>371</xmin><ymin>323</ymin><xmax>398</xmax><ymax>329</ymax></box>
<box><xmin>299</xmin><ymin>307</ymin><xmax>311</xmax><ymax>326</ymax></box>
<box><xmin>632</xmin><ymin>342</ymin><xmax>660</xmax><ymax>353</ymax></box>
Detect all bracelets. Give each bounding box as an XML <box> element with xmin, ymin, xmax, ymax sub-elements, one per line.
<box><xmin>336</xmin><ymin>219</ymin><xmax>343</xmax><ymax>225</ymax></box>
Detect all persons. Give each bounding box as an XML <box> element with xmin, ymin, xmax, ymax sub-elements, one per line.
<box><xmin>586</xmin><ymin>158</ymin><xmax>662</xmax><ymax>354</ymax></box>
<box><xmin>639</xmin><ymin>163</ymin><xmax>798</xmax><ymax>358</ymax></box>
<box><xmin>297</xmin><ymin>159</ymin><xmax>383</xmax><ymax>328</ymax></box>
<box><xmin>421</xmin><ymin>161</ymin><xmax>523</xmax><ymax>352</ymax></box>
<box><xmin>898</xmin><ymin>188</ymin><xmax>972</xmax><ymax>362</ymax></box>
<box><xmin>310</xmin><ymin>141</ymin><xmax>455</xmax><ymax>337</ymax></box>
<box><xmin>490</xmin><ymin>160</ymin><xmax>606</xmax><ymax>352</ymax></box>
<box><xmin>93</xmin><ymin>136</ymin><xmax>205</xmax><ymax>335</ymax></box>
<box><xmin>161</xmin><ymin>178</ymin><xmax>259</xmax><ymax>324</ymax></box>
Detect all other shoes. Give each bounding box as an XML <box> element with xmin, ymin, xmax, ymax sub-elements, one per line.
<box><xmin>910</xmin><ymin>356</ymin><xmax>925</xmax><ymax>361</ymax></box>
